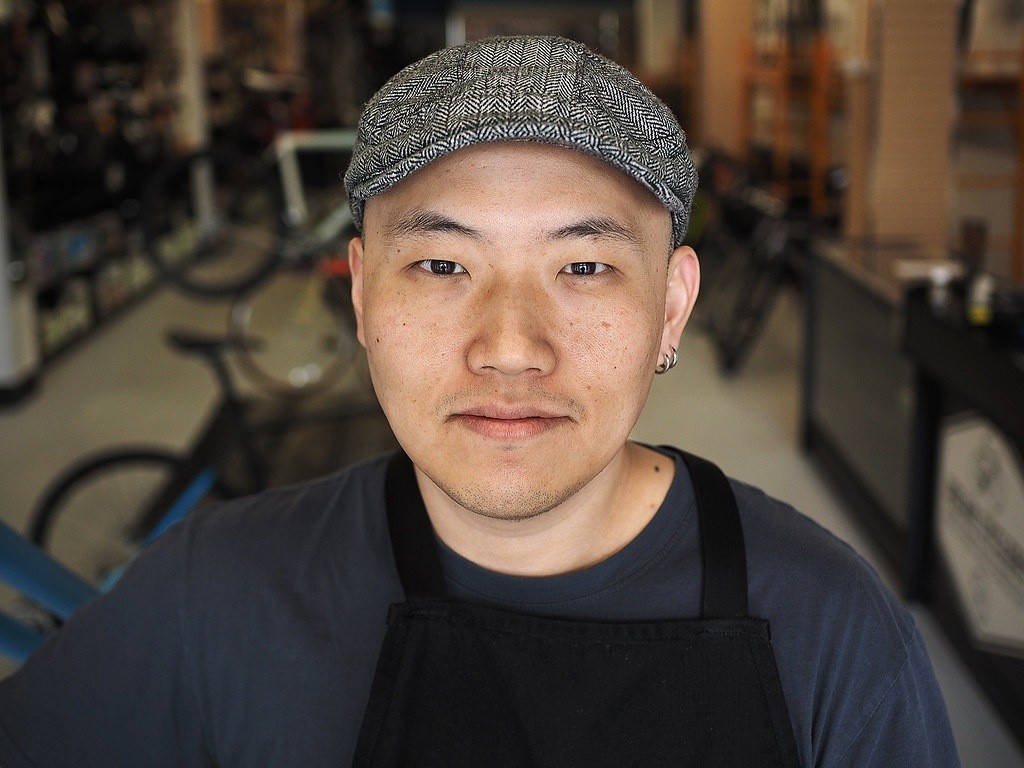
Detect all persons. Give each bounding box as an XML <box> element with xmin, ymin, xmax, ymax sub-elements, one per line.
<box><xmin>0</xmin><ymin>36</ymin><xmax>960</xmax><ymax>768</ymax></box>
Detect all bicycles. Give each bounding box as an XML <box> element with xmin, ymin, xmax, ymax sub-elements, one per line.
<box><xmin>26</xmin><ymin>135</ymin><xmax>387</xmax><ymax>636</ymax></box>
<box><xmin>695</xmin><ymin>153</ymin><xmax>805</xmax><ymax>373</ymax></box>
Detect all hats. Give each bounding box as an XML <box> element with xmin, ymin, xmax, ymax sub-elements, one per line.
<box><xmin>342</xmin><ymin>33</ymin><xmax>699</xmax><ymax>247</ymax></box>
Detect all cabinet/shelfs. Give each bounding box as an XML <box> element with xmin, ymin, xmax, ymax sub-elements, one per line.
<box><xmin>801</xmin><ymin>242</ymin><xmax>1024</xmax><ymax>606</ymax></box>
